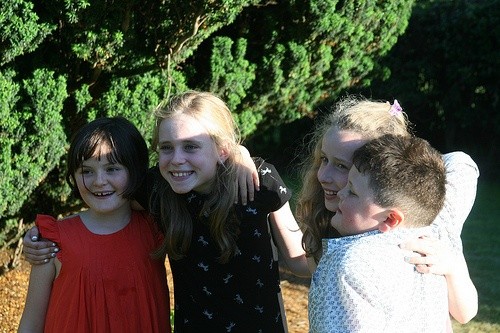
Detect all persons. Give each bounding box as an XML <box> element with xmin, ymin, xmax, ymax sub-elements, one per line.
<box><xmin>295</xmin><ymin>99</ymin><xmax>479</xmax><ymax>333</ymax></box>
<box><xmin>22</xmin><ymin>91</ymin><xmax>311</xmax><ymax>333</ymax></box>
<box><xmin>17</xmin><ymin>117</ymin><xmax>260</xmax><ymax>333</ymax></box>
